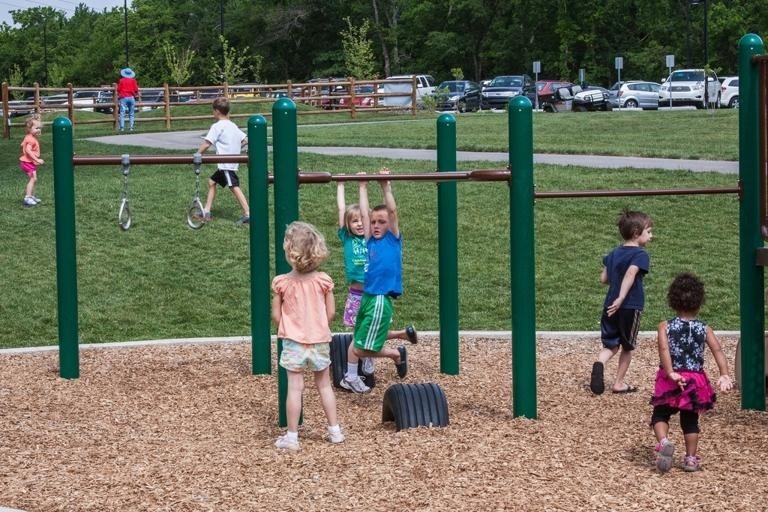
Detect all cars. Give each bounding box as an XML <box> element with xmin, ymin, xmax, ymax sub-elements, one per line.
<box><xmin>534</xmin><ymin>81</ymin><xmax>574</xmax><ymax>99</ymax></box>
<box><xmin>429</xmin><ymin>80</ymin><xmax>483</xmax><ymax>114</ymax></box>
<box><xmin>658</xmin><ymin>68</ymin><xmax>721</xmax><ymax>110</ymax></box>
<box><xmin>266</xmin><ymin>78</ymin><xmax>385</xmax><ymax>109</ymax></box>
<box><xmin>542</xmin><ymin>84</ymin><xmax>610</xmax><ymax>113</ymax></box>
<box><xmin>608</xmin><ymin>81</ymin><xmax>662</xmax><ymax>109</ymax></box>
<box><xmin>717</xmin><ymin>77</ymin><xmax>739</xmax><ymax>109</ymax></box>
<box><xmin>0</xmin><ymin>88</ymin><xmax>223</xmax><ymax>117</ymax></box>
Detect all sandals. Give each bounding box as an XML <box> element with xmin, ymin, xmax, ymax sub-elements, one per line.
<box><xmin>685</xmin><ymin>457</ymin><xmax>700</xmax><ymax>470</ymax></box>
<box><xmin>657</xmin><ymin>441</ymin><xmax>671</xmax><ymax>473</ymax></box>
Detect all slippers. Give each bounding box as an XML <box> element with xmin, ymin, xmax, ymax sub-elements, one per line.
<box><xmin>612</xmin><ymin>384</ymin><xmax>636</xmax><ymax>393</ymax></box>
<box><xmin>590</xmin><ymin>361</ymin><xmax>604</xmax><ymax>394</ymax></box>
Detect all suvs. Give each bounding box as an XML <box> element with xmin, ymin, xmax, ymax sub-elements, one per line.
<box><xmin>480</xmin><ymin>74</ymin><xmax>540</xmax><ymax>109</ymax></box>
<box><xmin>228</xmin><ymin>83</ymin><xmax>260</xmax><ymax>99</ymax></box>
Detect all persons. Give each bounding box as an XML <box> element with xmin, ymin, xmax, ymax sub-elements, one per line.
<box><xmin>196</xmin><ymin>97</ymin><xmax>250</xmax><ymax>225</ymax></box>
<box><xmin>17</xmin><ymin>117</ymin><xmax>44</xmax><ymax>205</ymax></box>
<box><xmin>351</xmin><ymin>165</ymin><xmax>418</xmax><ymax>378</ymax></box>
<box><xmin>587</xmin><ymin>210</ymin><xmax>651</xmax><ymax>397</ymax></box>
<box><xmin>117</xmin><ymin>67</ymin><xmax>138</xmax><ymax>131</ymax></box>
<box><xmin>649</xmin><ymin>271</ymin><xmax>734</xmax><ymax>473</ymax></box>
<box><xmin>334</xmin><ymin>168</ymin><xmax>381</xmax><ymax>395</ymax></box>
<box><xmin>270</xmin><ymin>218</ymin><xmax>344</xmax><ymax>447</ymax></box>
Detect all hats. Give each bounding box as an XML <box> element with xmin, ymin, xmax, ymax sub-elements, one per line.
<box><xmin>121</xmin><ymin>68</ymin><xmax>135</xmax><ymax>78</ymax></box>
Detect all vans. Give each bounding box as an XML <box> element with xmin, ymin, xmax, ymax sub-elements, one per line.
<box><xmin>383</xmin><ymin>74</ymin><xmax>439</xmax><ymax>107</ymax></box>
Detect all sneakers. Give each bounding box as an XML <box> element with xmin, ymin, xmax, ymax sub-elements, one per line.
<box><xmin>276</xmin><ymin>436</ymin><xmax>298</xmax><ymax>450</ymax></box>
<box><xmin>23</xmin><ymin>195</ymin><xmax>39</xmax><ymax>205</ymax></box>
<box><xmin>360</xmin><ymin>357</ymin><xmax>375</xmax><ymax>375</ymax></box>
<box><xmin>195</xmin><ymin>211</ymin><xmax>211</xmax><ymax>220</ymax></box>
<box><xmin>329</xmin><ymin>432</ymin><xmax>346</xmax><ymax>442</ymax></box>
<box><xmin>236</xmin><ymin>215</ymin><xmax>250</xmax><ymax>222</ymax></box>
<box><xmin>395</xmin><ymin>346</ymin><xmax>407</xmax><ymax>379</ymax></box>
<box><xmin>407</xmin><ymin>327</ymin><xmax>419</xmax><ymax>344</ymax></box>
<box><xmin>340</xmin><ymin>374</ymin><xmax>371</xmax><ymax>394</ymax></box>
